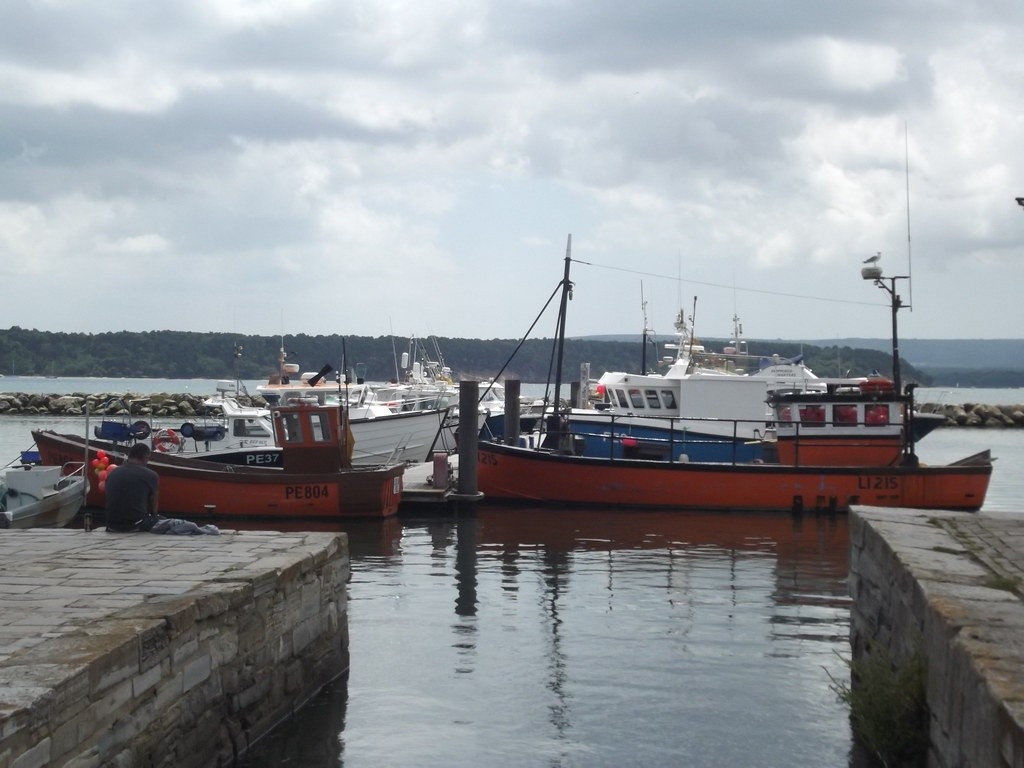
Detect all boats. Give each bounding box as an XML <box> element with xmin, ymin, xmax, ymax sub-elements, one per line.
<box><xmin>0</xmin><ymin>461</ymin><xmax>91</xmax><ymax>527</ymax></box>
<box><xmin>29</xmin><ymin>397</ymin><xmax>406</xmax><ymax>518</ymax></box>
<box><xmin>155</xmin><ymin>233</ymin><xmax>998</xmax><ymax>513</ymax></box>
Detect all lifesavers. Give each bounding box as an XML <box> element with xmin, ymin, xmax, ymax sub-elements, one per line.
<box><xmin>154</xmin><ymin>429</ymin><xmax>180</xmax><ymax>453</ymax></box>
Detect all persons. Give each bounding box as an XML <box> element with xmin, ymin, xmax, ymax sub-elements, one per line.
<box><xmin>104</xmin><ymin>443</ymin><xmax>168</xmax><ymax>532</ymax></box>
